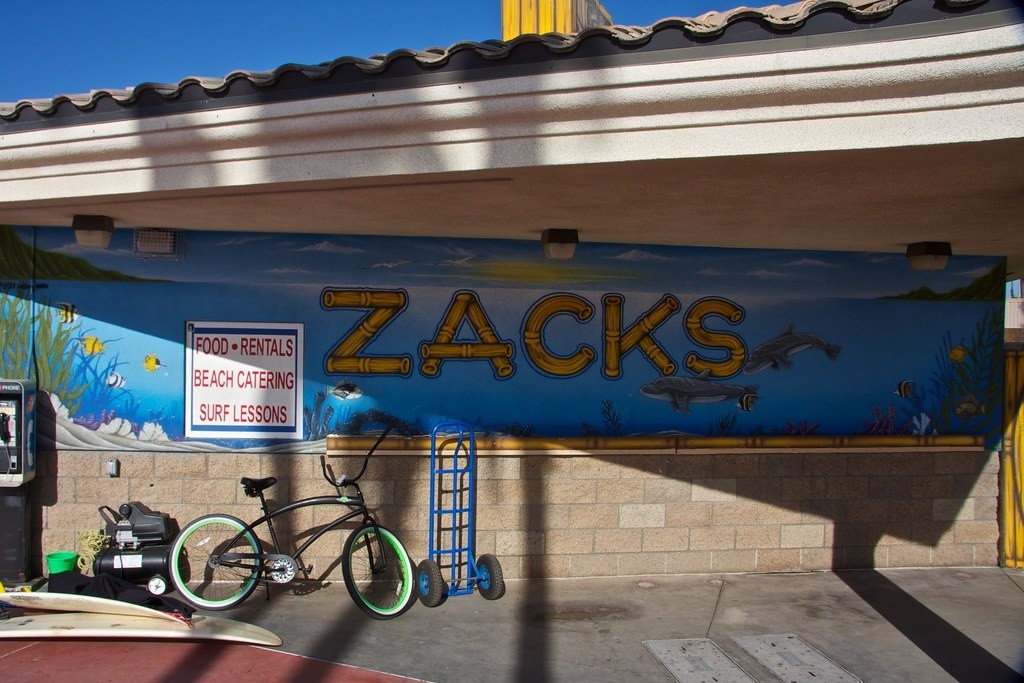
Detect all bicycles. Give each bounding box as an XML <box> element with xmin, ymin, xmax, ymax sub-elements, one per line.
<box><xmin>166</xmin><ymin>426</ymin><xmax>414</xmax><ymax>621</ymax></box>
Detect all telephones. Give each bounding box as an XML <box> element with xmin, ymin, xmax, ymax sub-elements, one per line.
<box><xmin>0</xmin><ymin>400</ymin><xmax>20</xmax><ymax>450</ymax></box>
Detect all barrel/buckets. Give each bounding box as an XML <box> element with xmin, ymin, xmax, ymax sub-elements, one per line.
<box><xmin>45</xmin><ymin>551</ymin><xmax>78</xmax><ymax>574</ymax></box>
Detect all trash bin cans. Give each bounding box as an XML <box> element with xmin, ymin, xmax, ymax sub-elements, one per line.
<box><xmin>45</xmin><ymin>552</ymin><xmax>78</xmax><ymax>574</ymax></box>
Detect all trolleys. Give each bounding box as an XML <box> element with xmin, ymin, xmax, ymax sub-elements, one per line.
<box><xmin>415</xmin><ymin>422</ymin><xmax>503</xmax><ymax>608</ymax></box>
